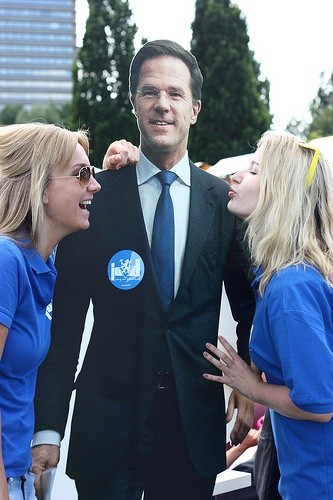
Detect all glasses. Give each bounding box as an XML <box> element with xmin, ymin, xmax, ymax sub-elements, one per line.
<box><xmin>299</xmin><ymin>142</ymin><xmax>321</xmax><ymax>190</ymax></box>
<box><xmin>48</xmin><ymin>166</ymin><xmax>96</xmax><ymax>188</ymax></box>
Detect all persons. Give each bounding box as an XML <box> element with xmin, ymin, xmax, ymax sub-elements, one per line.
<box><xmin>0</xmin><ymin>121</ymin><xmax>139</xmax><ymax>500</ymax></box>
<box><xmin>201</xmin><ymin>129</ymin><xmax>333</xmax><ymax>500</ymax></box>
<box><xmin>29</xmin><ymin>40</ymin><xmax>267</xmax><ymax>500</ymax></box>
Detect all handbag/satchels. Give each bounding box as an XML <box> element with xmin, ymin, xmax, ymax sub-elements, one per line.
<box><xmin>253</xmin><ymin>406</ymin><xmax>282</xmax><ymax>500</ymax></box>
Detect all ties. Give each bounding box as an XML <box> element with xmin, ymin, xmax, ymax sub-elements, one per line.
<box><xmin>151</xmin><ymin>168</ymin><xmax>178</xmax><ymax>311</ymax></box>
